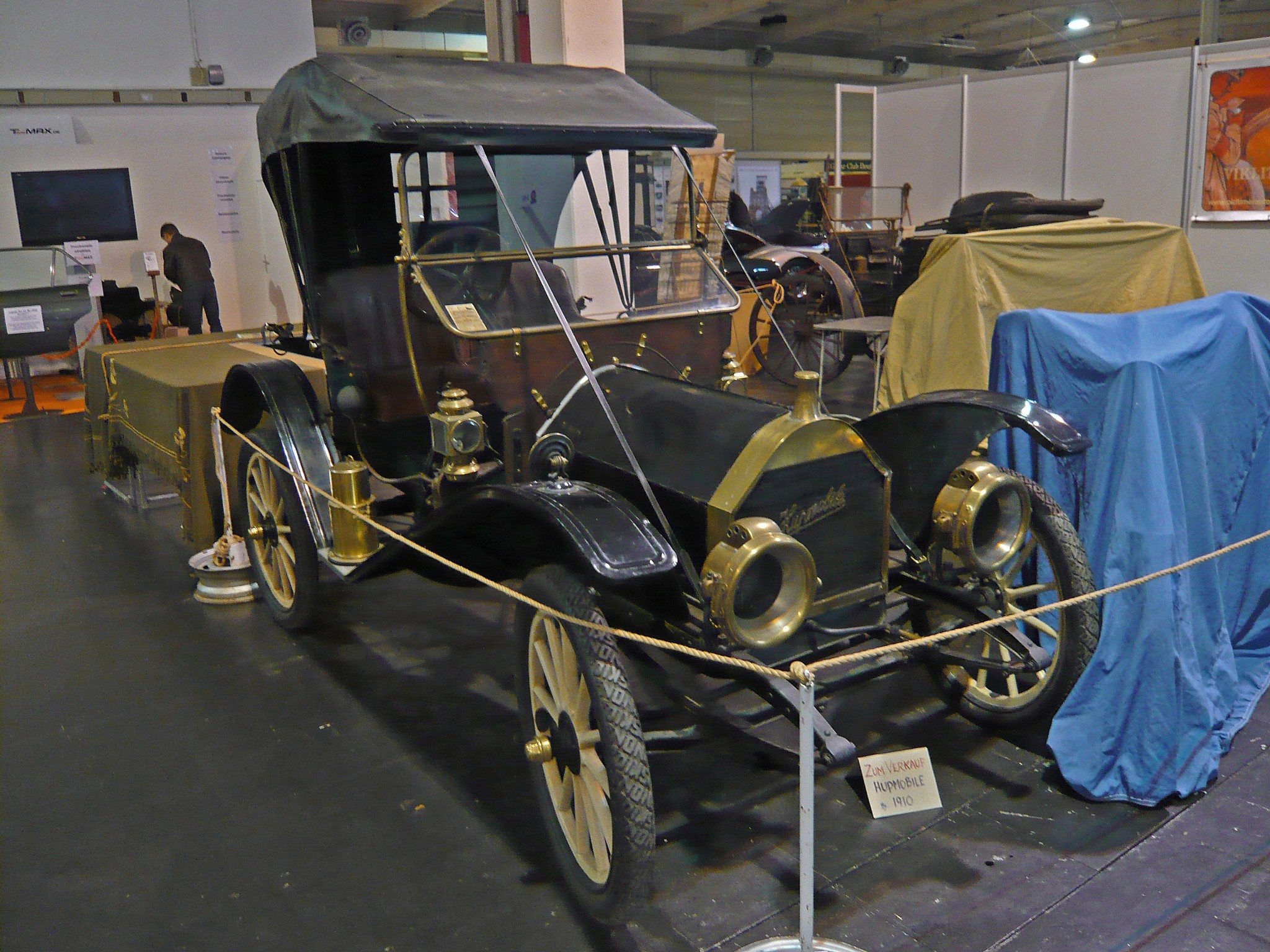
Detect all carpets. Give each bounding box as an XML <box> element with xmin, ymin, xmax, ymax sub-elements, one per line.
<box><xmin>0</xmin><ymin>376</ymin><xmax>86</xmax><ymax>423</ymax></box>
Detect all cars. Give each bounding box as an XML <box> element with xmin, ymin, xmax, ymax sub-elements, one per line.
<box><xmin>207</xmin><ymin>38</ymin><xmax>1107</xmax><ymax>935</ymax></box>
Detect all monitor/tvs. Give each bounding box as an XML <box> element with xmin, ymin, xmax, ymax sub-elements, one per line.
<box><xmin>11</xmin><ymin>168</ymin><xmax>138</xmax><ymax>248</ymax></box>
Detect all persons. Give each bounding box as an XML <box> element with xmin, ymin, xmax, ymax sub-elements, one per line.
<box><xmin>161</xmin><ymin>222</ymin><xmax>223</xmax><ymax>335</ymax></box>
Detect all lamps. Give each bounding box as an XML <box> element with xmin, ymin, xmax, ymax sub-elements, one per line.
<box><xmin>746</xmin><ymin>45</ymin><xmax>775</xmax><ymax>68</ymax></box>
<box><xmin>881</xmin><ymin>55</ymin><xmax>910</xmax><ymax>78</ymax></box>
<box><xmin>1067</xmin><ymin>14</ymin><xmax>1093</xmax><ymax>32</ymax></box>
<box><xmin>1075</xmin><ymin>49</ymin><xmax>1099</xmax><ymax>67</ymax></box>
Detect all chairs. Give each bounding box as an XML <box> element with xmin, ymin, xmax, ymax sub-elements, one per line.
<box><xmin>410</xmin><ymin>225</ymin><xmax>581</xmax><ymax>324</ymax></box>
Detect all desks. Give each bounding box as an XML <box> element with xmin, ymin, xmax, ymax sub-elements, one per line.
<box><xmin>78</xmin><ymin>322</ymin><xmax>332</xmax><ymax>551</ymax></box>
<box><xmin>813</xmin><ymin>316</ymin><xmax>894</xmax><ymax>421</ymax></box>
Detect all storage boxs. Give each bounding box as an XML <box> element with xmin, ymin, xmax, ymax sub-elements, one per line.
<box><xmin>138</xmin><ymin>306</ymin><xmax>187</xmax><ymax>340</ymax></box>
